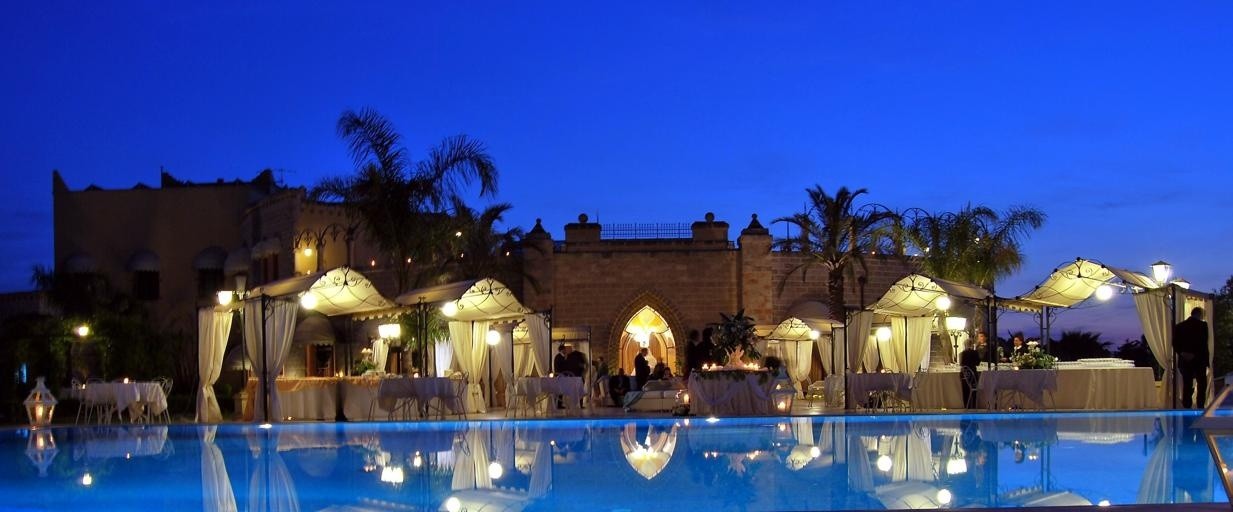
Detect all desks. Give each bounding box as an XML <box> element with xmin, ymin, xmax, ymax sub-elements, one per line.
<box><xmin>66</xmin><ymin>348</ymin><xmax>1168</xmax><ymax>429</ymax></box>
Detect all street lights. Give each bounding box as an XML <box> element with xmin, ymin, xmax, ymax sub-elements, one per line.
<box><xmin>216</xmin><ymin>288</ymin><xmax>249</xmax><ymax>391</ymax></box>
<box><xmin>1150</xmin><ymin>260</ymin><xmax>1173</xmax><ymax>378</ymax></box>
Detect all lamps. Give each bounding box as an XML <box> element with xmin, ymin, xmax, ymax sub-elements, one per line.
<box><xmin>934</xmin><ymin>292</ymin><xmax>950</xmax><ymax>311</ymax></box>
<box><xmin>440</xmin><ymin>299</ymin><xmax>460</xmax><ymax>320</ymax></box>
<box><xmin>484</xmin><ymin>325</ymin><xmax>503</xmax><ymax>347</ymax></box>
<box><xmin>1094</xmin><ymin>282</ymin><xmax>1113</xmax><ymax>303</ymax></box>
<box><xmin>15</xmin><ymin>421</ymin><xmax>1233</xmax><ymax>512</ymax></box>
<box><xmin>874</xmin><ymin>323</ymin><xmax>892</xmax><ymax>343</ymax></box>
<box><xmin>19</xmin><ymin>374</ymin><xmax>60</xmax><ymax>427</ymax></box>
<box><xmin>297</xmin><ymin>289</ymin><xmax>318</xmax><ymax>314</ymax></box>
<box><xmin>807</xmin><ymin>328</ymin><xmax>821</xmax><ymax>342</ymax></box>
<box><xmin>375</xmin><ymin>318</ymin><xmax>402</xmax><ymax>344</ymax></box>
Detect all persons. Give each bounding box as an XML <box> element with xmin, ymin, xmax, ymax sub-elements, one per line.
<box><xmin>696</xmin><ymin>327</ymin><xmax>722</xmax><ymax>366</ymax></box>
<box><xmin>975</xmin><ymin>332</ymin><xmax>993</xmax><ymax>363</ymax></box>
<box><xmin>959</xmin><ymin>339</ymin><xmax>982</xmax><ymax>409</ymax></box>
<box><xmin>1172</xmin><ymin>307</ymin><xmax>1210</xmax><ymax>409</ymax></box>
<box><xmin>682</xmin><ymin>330</ymin><xmax>701</xmax><ymax>380</ymax></box>
<box><xmin>554</xmin><ymin>346</ymin><xmax>674</xmax><ymax>409</ymax></box>
<box><xmin>1000</xmin><ymin>335</ymin><xmax>1029</xmax><ymax>363</ymax></box>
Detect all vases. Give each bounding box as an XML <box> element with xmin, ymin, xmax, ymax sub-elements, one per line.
<box><xmin>719</xmin><ymin>342</ymin><xmax>747</xmax><ymax>371</ymax></box>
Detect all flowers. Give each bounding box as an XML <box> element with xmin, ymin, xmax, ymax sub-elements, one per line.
<box><xmin>1008</xmin><ymin>341</ymin><xmax>1062</xmax><ymax>370</ymax></box>
<box><xmin>706</xmin><ymin>305</ymin><xmax>765</xmax><ymax>361</ymax></box>
